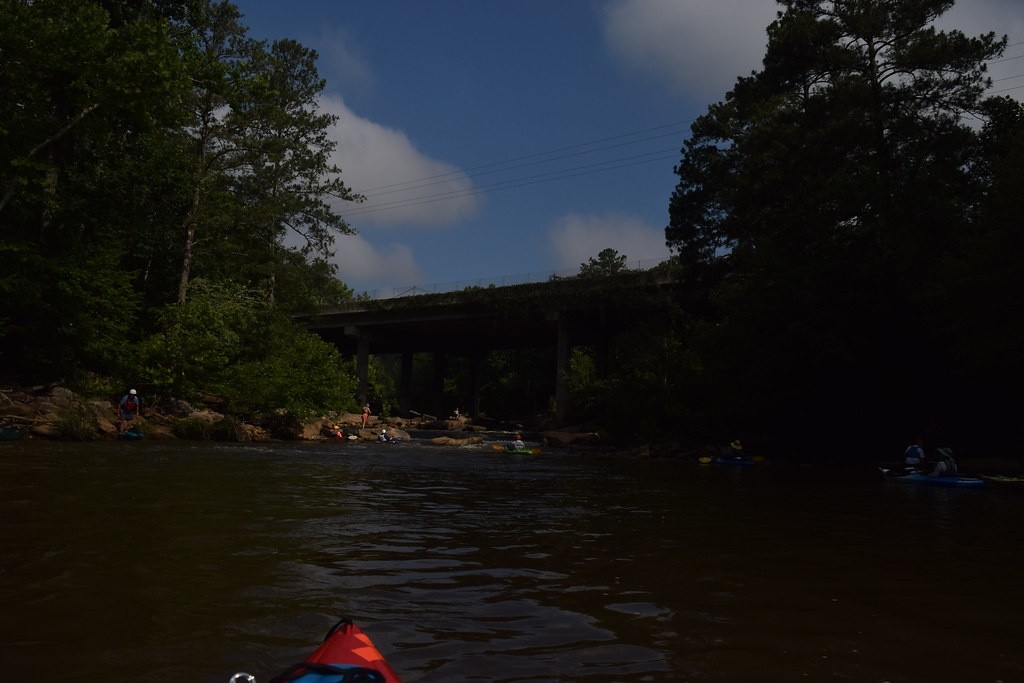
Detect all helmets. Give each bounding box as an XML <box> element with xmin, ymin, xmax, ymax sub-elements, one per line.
<box><xmin>382</xmin><ymin>429</ymin><xmax>387</xmax><ymax>434</ymax></box>
<box><xmin>515</xmin><ymin>435</ymin><xmax>521</xmax><ymax>440</ymax></box>
<box><xmin>915</xmin><ymin>438</ymin><xmax>926</xmax><ymax>447</ymax></box>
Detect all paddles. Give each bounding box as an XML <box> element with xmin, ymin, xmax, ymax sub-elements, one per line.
<box><xmin>699</xmin><ymin>456</ymin><xmax>766</xmax><ymax>464</ymax></box>
<box><xmin>493</xmin><ymin>444</ymin><xmax>541</xmax><ymax>454</ymax></box>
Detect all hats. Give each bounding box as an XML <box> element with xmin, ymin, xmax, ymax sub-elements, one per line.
<box><xmin>366</xmin><ymin>403</ymin><xmax>370</xmax><ymax>406</ymax></box>
<box><xmin>937</xmin><ymin>447</ymin><xmax>955</xmax><ymax>462</ymax></box>
<box><xmin>730</xmin><ymin>440</ymin><xmax>743</xmax><ymax>450</ymax></box>
<box><xmin>130</xmin><ymin>388</ymin><xmax>137</xmax><ymax>395</ymax></box>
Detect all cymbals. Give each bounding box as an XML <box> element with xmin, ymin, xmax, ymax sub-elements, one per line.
<box><xmin>960</xmin><ymin>467</ymin><xmax>1023</xmax><ymax>483</ymax></box>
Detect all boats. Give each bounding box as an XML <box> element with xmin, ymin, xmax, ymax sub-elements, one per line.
<box><xmin>879</xmin><ymin>464</ymin><xmax>989</xmax><ymax>489</ymax></box>
<box><xmin>119</xmin><ymin>428</ymin><xmax>139</xmax><ymax>441</ymax></box>
<box><xmin>376</xmin><ymin>440</ymin><xmax>399</xmax><ymax>444</ymax></box>
<box><xmin>430</xmin><ymin>436</ymin><xmax>483</xmax><ymax>446</ymax></box>
<box><xmin>493</xmin><ymin>444</ymin><xmax>540</xmax><ymax>455</ymax></box>
<box><xmin>699</xmin><ymin>453</ymin><xmax>766</xmax><ymax>466</ymax></box>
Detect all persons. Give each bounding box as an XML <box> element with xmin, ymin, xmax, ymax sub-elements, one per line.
<box><xmin>512</xmin><ymin>432</ymin><xmax>521</xmax><ymax>440</ymax></box>
<box><xmin>902</xmin><ymin>438</ymin><xmax>925</xmax><ymax>474</ymax></box>
<box><xmin>378</xmin><ymin>429</ymin><xmax>393</xmax><ymax>442</ymax></box>
<box><xmin>362</xmin><ymin>403</ymin><xmax>372</xmax><ymax>429</ymax></box>
<box><xmin>720</xmin><ymin>440</ymin><xmax>744</xmax><ymax>460</ymax></box>
<box><xmin>117</xmin><ymin>389</ymin><xmax>139</xmax><ymax>432</ymax></box>
<box><xmin>928</xmin><ymin>448</ymin><xmax>957</xmax><ymax>478</ymax></box>
